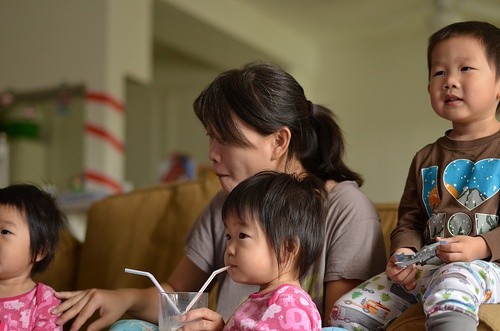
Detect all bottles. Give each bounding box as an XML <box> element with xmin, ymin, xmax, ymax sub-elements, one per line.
<box><xmin>11</xmin><ymin>101</ymin><xmax>50</xmax><ymax>187</ymax></box>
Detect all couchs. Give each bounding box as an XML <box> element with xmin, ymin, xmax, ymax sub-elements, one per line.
<box><xmin>32</xmin><ymin>172</ymin><xmax>500</xmax><ymax>331</ymax></box>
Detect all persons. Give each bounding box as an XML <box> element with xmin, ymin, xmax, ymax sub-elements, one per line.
<box><xmin>1</xmin><ymin>184</ymin><xmax>63</xmax><ymax>331</ymax></box>
<box><xmin>52</xmin><ymin>65</ymin><xmax>388</xmax><ymax>331</ymax></box>
<box><xmin>180</xmin><ymin>173</ymin><xmax>322</xmax><ymax>330</ymax></box>
<box><xmin>329</xmin><ymin>21</ymin><xmax>500</xmax><ymax>331</ymax></box>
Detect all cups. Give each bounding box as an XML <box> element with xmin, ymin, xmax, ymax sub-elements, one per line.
<box><xmin>159</xmin><ymin>291</ymin><xmax>209</xmax><ymax>331</ymax></box>
